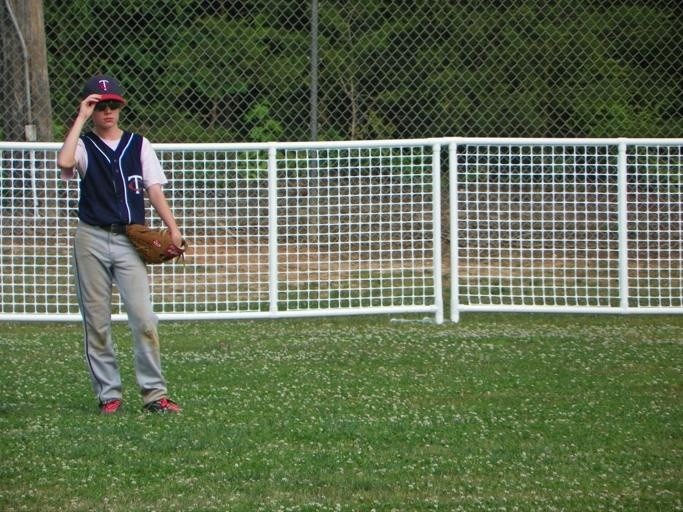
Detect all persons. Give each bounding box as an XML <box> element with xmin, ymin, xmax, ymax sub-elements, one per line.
<box><xmin>56</xmin><ymin>75</ymin><xmax>187</xmax><ymax>413</ymax></box>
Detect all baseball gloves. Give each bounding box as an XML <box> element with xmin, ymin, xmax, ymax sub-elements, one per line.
<box><xmin>128</xmin><ymin>223</ymin><xmax>187</xmax><ymax>265</ymax></box>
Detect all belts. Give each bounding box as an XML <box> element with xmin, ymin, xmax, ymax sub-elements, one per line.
<box><xmin>99</xmin><ymin>222</ymin><xmax>123</xmax><ymax>233</ymax></box>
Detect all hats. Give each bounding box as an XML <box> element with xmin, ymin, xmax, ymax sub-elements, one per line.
<box><xmin>83</xmin><ymin>75</ymin><xmax>125</xmax><ymax>103</ymax></box>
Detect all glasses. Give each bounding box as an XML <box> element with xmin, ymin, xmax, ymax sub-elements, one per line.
<box><xmin>95</xmin><ymin>100</ymin><xmax>120</xmax><ymax>110</ymax></box>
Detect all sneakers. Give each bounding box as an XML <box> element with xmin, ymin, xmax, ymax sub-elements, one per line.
<box><xmin>146</xmin><ymin>398</ymin><xmax>183</xmax><ymax>414</ymax></box>
<box><xmin>100</xmin><ymin>399</ymin><xmax>121</xmax><ymax>414</ymax></box>
